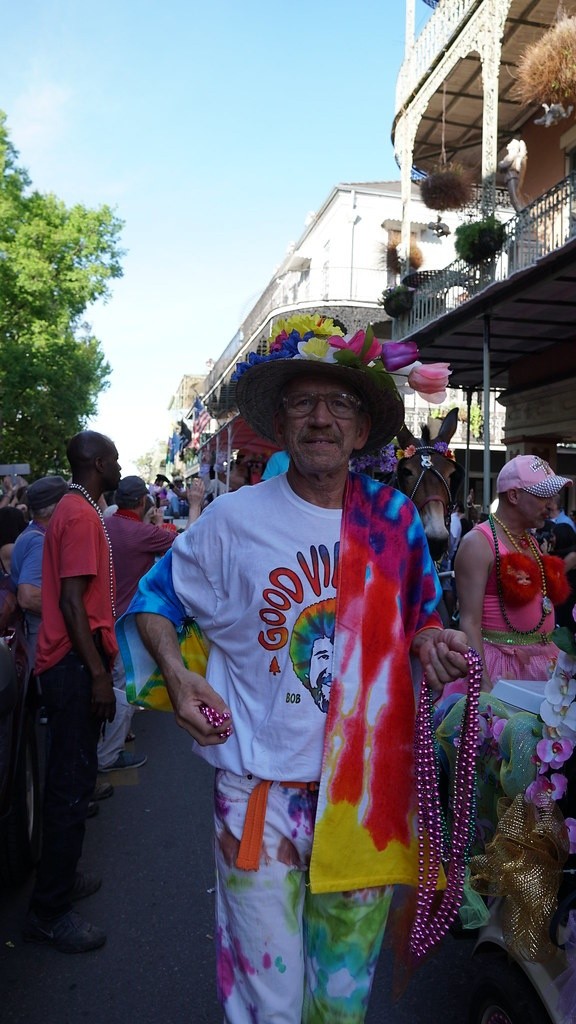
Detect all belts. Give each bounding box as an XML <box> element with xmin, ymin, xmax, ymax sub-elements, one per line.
<box><xmin>236</xmin><ymin>778</ymin><xmax>320</xmax><ymax>873</ymax></box>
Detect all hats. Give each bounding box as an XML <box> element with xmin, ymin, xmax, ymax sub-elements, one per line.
<box><xmin>496</xmin><ymin>455</ymin><xmax>573</xmax><ymax>498</ymax></box>
<box><xmin>26</xmin><ymin>476</ymin><xmax>69</xmax><ymax>510</ymax></box>
<box><xmin>116</xmin><ymin>475</ymin><xmax>150</xmax><ymax>500</ymax></box>
<box><xmin>231</xmin><ymin>314</ymin><xmax>452</xmax><ymax>458</ymax></box>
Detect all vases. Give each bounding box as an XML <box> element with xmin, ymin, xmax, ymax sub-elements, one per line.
<box><xmin>384</xmin><ymin>291</ymin><xmax>414</xmax><ymax>317</ymax></box>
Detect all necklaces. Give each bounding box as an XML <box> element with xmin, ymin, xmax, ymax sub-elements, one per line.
<box><xmin>487</xmin><ymin>514</ymin><xmax>548</xmax><ymax>635</ymax></box>
<box><xmin>113</xmin><ymin>514</ymin><xmax>139</xmax><ymax>521</ymax></box>
<box><xmin>199</xmin><ymin>705</ymin><xmax>232</xmax><ymax>740</ymax></box>
<box><xmin>32</xmin><ymin>521</ymin><xmax>46</xmax><ymax>531</ymax></box>
<box><xmin>409</xmin><ymin>645</ymin><xmax>483</xmax><ymax>956</ymax></box>
<box><xmin>69</xmin><ymin>483</ymin><xmax>116</xmax><ymax>617</ymax></box>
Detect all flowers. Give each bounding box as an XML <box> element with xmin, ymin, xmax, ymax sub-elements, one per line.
<box><xmin>377</xmin><ymin>285</ymin><xmax>416</xmax><ymax>307</ymax></box>
<box><xmin>229</xmin><ymin>314</ymin><xmax>454</xmax><ymax>404</ymax></box>
<box><xmin>378</xmin><ymin>442</ymin><xmax>455</xmax><ymax>474</ymax></box>
<box><xmin>525</xmin><ymin>646</ymin><xmax>576</xmax><ymax>859</ymax></box>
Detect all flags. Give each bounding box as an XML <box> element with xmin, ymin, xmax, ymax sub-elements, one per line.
<box><xmin>165</xmin><ymin>396</ymin><xmax>213</xmax><ymax>464</ymax></box>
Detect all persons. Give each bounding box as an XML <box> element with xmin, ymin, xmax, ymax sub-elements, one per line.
<box><xmin>87</xmin><ymin>451</ymin><xmax>289</xmax><ymax>818</ymax></box>
<box><xmin>0</xmin><ymin>473</ymin><xmax>69</xmax><ymax>666</ymax></box>
<box><xmin>531</xmin><ymin>495</ymin><xmax>576</xmax><ymax>635</ymax></box>
<box><xmin>21</xmin><ymin>430</ymin><xmax>120</xmax><ymax>954</ymax></box>
<box><xmin>498</xmin><ymin>138</ymin><xmax>524</xmax><ymax>212</ymax></box>
<box><xmin>443</xmin><ymin>455</ymin><xmax>576</xmax><ymax>696</ymax></box>
<box><xmin>439</xmin><ymin>495</ymin><xmax>489</xmax><ymax>629</ymax></box>
<box><xmin>114</xmin><ymin>314</ymin><xmax>468</xmax><ymax>1024</ymax></box>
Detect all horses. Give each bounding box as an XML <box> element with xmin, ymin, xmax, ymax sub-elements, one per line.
<box><xmin>380</xmin><ymin>407</ymin><xmax>459</xmax><ymax>561</ymax></box>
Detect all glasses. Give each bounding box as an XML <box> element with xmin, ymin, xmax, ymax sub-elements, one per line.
<box><xmin>280</xmin><ymin>391</ymin><xmax>363</xmax><ymax>419</ymax></box>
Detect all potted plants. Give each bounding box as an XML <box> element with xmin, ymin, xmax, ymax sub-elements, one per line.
<box><xmin>452</xmin><ymin>214</ymin><xmax>508</xmax><ymax>267</ymax></box>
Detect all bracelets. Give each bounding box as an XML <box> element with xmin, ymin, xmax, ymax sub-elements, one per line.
<box><xmin>5</xmin><ymin>494</ymin><xmax>11</xmax><ymax>499</ymax></box>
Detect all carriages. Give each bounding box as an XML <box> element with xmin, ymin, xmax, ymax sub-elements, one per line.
<box><xmin>193</xmin><ymin>411</ymin><xmax>466</xmax><ymax>561</ymax></box>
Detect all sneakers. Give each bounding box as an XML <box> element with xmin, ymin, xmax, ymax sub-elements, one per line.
<box><xmin>24</xmin><ymin>910</ymin><xmax>105</xmax><ymax>954</ymax></box>
<box><xmin>91</xmin><ymin>783</ymin><xmax>113</xmax><ymax>800</ymax></box>
<box><xmin>101</xmin><ymin>751</ymin><xmax>147</xmax><ymax>773</ymax></box>
<box><xmin>71</xmin><ymin>872</ymin><xmax>102</xmax><ymax>901</ymax></box>
<box><xmin>86</xmin><ymin>802</ymin><xmax>99</xmax><ymax>819</ymax></box>
